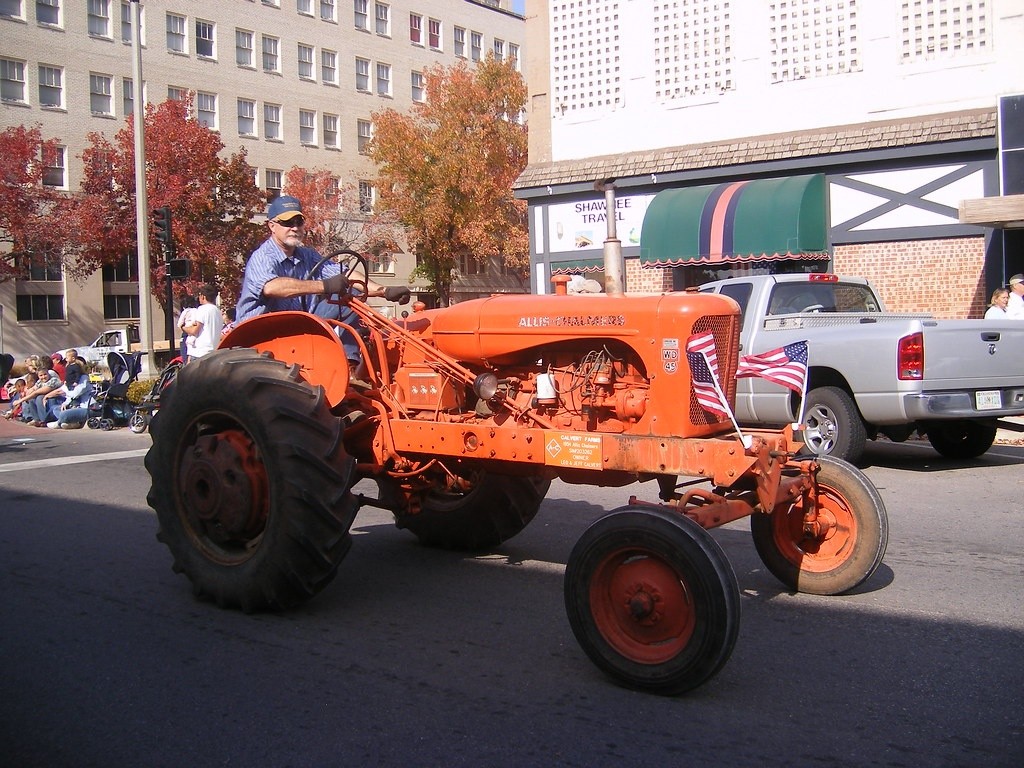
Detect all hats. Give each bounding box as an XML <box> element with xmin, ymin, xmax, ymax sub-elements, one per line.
<box><xmin>1010</xmin><ymin>274</ymin><xmax>1024</xmax><ymax>285</ymax></box>
<box><xmin>197</xmin><ymin>284</ymin><xmax>215</xmax><ymax>298</ymax></box>
<box><xmin>50</xmin><ymin>353</ymin><xmax>63</xmax><ymax>361</ymax></box>
<box><xmin>268</xmin><ymin>196</ymin><xmax>305</xmax><ymax>222</ymax></box>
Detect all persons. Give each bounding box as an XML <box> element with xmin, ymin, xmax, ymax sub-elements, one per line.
<box><xmin>1006</xmin><ymin>274</ymin><xmax>1024</xmax><ymax>319</ymax></box>
<box><xmin>984</xmin><ymin>288</ymin><xmax>1010</xmax><ymax>320</ymax></box>
<box><xmin>235</xmin><ymin>196</ymin><xmax>410</xmax><ymax>391</ymax></box>
<box><xmin>0</xmin><ymin>286</ymin><xmax>237</xmax><ymax>430</ymax></box>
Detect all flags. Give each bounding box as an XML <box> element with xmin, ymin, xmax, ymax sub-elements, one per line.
<box><xmin>685</xmin><ymin>329</ymin><xmax>733</xmax><ymax>418</ymax></box>
<box><xmin>734</xmin><ymin>339</ymin><xmax>809</xmax><ymax>397</ymax></box>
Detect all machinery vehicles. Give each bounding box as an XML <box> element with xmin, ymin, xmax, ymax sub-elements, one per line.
<box><xmin>145</xmin><ymin>249</ymin><xmax>891</xmax><ymax>701</ymax></box>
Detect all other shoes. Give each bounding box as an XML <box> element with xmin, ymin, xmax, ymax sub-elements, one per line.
<box><xmin>332</xmin><ymin>402</ymin><xmax>368</xmax><ymax>425</ymax></box>
<box><xmin>34</xmin><ymin>421</ymin><xmax>46</xmax><ymax>427</ymax></box>
<box><xmin>348</xmin><ymin>361</ymin><xmax>372</xmax><ymax>392</ymax></box>
<box><xmin>26</xmin><ymin>419</ymin><xmax>36</xmax><ymax>426</ymax></box>
<box><xmin>47</xmin><ymin>421</ymin><xmax>61</xmax><ymax>429</ymax></box>
<box><xmin>61</xmin><ymin>422</ymin><xmax>80</xmax><ymax>429</ymax></box>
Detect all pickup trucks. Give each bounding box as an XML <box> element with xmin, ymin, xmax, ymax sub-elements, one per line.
<box><xmin>55</xmin><ymin>321</ymin><xmax>181</xmax><ymax>375</ymax></box>
<box><xmin>695</xmin><ymin>272</ymin><xmax>1024</xmax><ymax>474</ymax></box>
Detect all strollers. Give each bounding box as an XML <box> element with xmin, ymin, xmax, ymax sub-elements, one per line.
<box><xmin>86</xmin><ymin>351</ymin><xmax>141</xmax><ymax>432</ymax></box>
<box><xmin>128</xmin><ymin>356</ymin><xmax>186</xmax><ymax>434</ymax></box>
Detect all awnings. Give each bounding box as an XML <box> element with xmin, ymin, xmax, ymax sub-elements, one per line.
<box><xmin>640</xmin><ymin>173</ymin><xmax>831</xmax><ymax>268</ymax></box>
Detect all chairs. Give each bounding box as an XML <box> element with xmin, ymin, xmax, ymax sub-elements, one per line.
<box><xmin>783</xmin><ymin>293</ymin><xmax>817</xmax><ymax>311</ymax></box>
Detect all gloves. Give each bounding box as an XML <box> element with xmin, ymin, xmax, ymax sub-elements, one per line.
<box><xmin>384</xmin><ymin>285</ymin><xmax>411</xmax><ymax>305</ymax></box>
<box><xmin>323</xmin><ymin>274</ymin><xmax>349</xmax><ymax>295</ymax></box>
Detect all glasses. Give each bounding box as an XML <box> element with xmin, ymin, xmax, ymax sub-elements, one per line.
<box><xmin>273</xmin><ymin>217</ymin><xmax>304</xmax><ymax>227</ymax></box>
